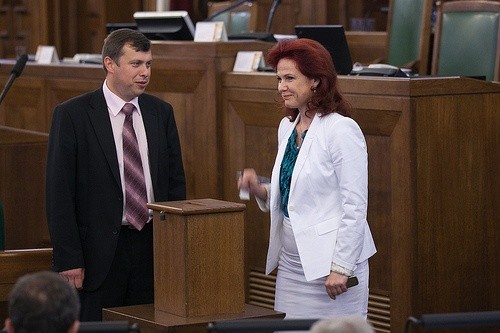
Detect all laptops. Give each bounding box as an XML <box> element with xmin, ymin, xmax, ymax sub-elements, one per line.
<box><xmin>296</xmin><ymin>23</ymin><xmax>384</xmax><ymax>76</ymax></box>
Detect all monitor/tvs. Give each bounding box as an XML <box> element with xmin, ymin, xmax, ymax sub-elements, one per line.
<box><xmin>134</xmin><ymin>11</ymin><xmax>196</xmax><ymax>43</ymax></box>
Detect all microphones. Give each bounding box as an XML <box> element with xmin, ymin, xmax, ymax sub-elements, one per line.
<box><xmin>106</xmin><ymin>22</ymin><xmax>138</xmax><ymax>35</ymax></box>
<box><xmin>0</xmin><ymin>54</ymin><xmax>28</xmax><ymax>102</ymax></box>
<box><xmin>228</xmin><ymin>0</ymin><xmax>283</xmax><ymax>42</ymax></box>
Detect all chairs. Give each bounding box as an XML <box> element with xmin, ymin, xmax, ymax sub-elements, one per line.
<box><xmin>207</xmin><ymin>1</ymin><xmax>257</xmax><ymax>38</ymax></box>
<box><xmin>383</xmin><ymin>0</ymin><xmax>500</xmax><ymax>84</ymax></box>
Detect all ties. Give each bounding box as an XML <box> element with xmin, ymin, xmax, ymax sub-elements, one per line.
<box><xmin>121</xmin><ymin>103</ymin><xmax>150</xmax><ymax>232</ymax></box>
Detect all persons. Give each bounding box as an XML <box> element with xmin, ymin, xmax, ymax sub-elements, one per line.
<box><xmin>236</xmin><ymin>38</ymin><xmax>378</xmax><ymax>320</ymax></box>
<box><xmin>46</xmin><ymin>29</ymin><xmax>186</xmax><ymax>322</ymax></box>
<box><xmin>3</xmin><ymin>271</ymin><xmax>82</xmax><ymax>333</ymax></box>
